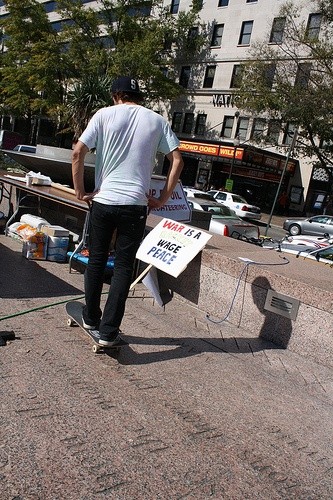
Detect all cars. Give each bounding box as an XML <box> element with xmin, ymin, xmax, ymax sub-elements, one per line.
<box><xmin>278</xmin><ymin>235</ymin><xmax>333</xmax><ymax>265</ymax></box>
<box><xmin>206</xmin><ymin>189</ymin><xmax>262</xmax><ymax>222</ymax></box>
<box><xmin>12</xmin><ymin>144</ymin><xmax>37</xmax><ymax>154</ymax></box>
<box><xmin>185</xmin><ymin>197</ymin><xmax>260</xmax><ymax>241</ymax></box>
<box><xmin>182</xmin><ymin>186</ymin><xmax>218</xmax><ymax>203</ymax></box>
<box><xmin>282</xmin><ymin>214</ymin><xmax>333</xmax><ymax>236</ymax></box>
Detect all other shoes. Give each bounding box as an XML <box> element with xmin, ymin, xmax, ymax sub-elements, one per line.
<box><xmin>82</xmin><ymin>315</ymin><xmax>96</xmax><ymax>329</ymax></box>
<box><xmin>98</xmin><ymin>332</ymin><xmax>121</xmax><ymax>347</ymax></box>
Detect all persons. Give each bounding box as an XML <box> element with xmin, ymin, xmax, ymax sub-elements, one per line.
<box><xmin>185</xmin><ymin>182</ymin><xmax>229</xmax><ymax>192</ymax></box>
<box><xmin>70</xmin><ymin>74</ymin><xmax>184</xmax><ymax>346</ymax></box>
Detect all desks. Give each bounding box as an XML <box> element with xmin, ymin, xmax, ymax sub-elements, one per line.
<box><xmin>0</xmin><ymin>176</ymin><xmax>93</xmax><ymax>276</ymax></box>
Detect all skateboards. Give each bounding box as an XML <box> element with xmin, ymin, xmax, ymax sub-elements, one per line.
<box><xmin>65</xmin><ymin>301</ymin><xmax>128</xmax><ymax>354</ymax></box>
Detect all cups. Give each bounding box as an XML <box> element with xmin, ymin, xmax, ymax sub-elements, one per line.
<box><xmin>26</xmin><ymin>176</ymin><xmax>33</xmax><ymax>188</ymax></box>
